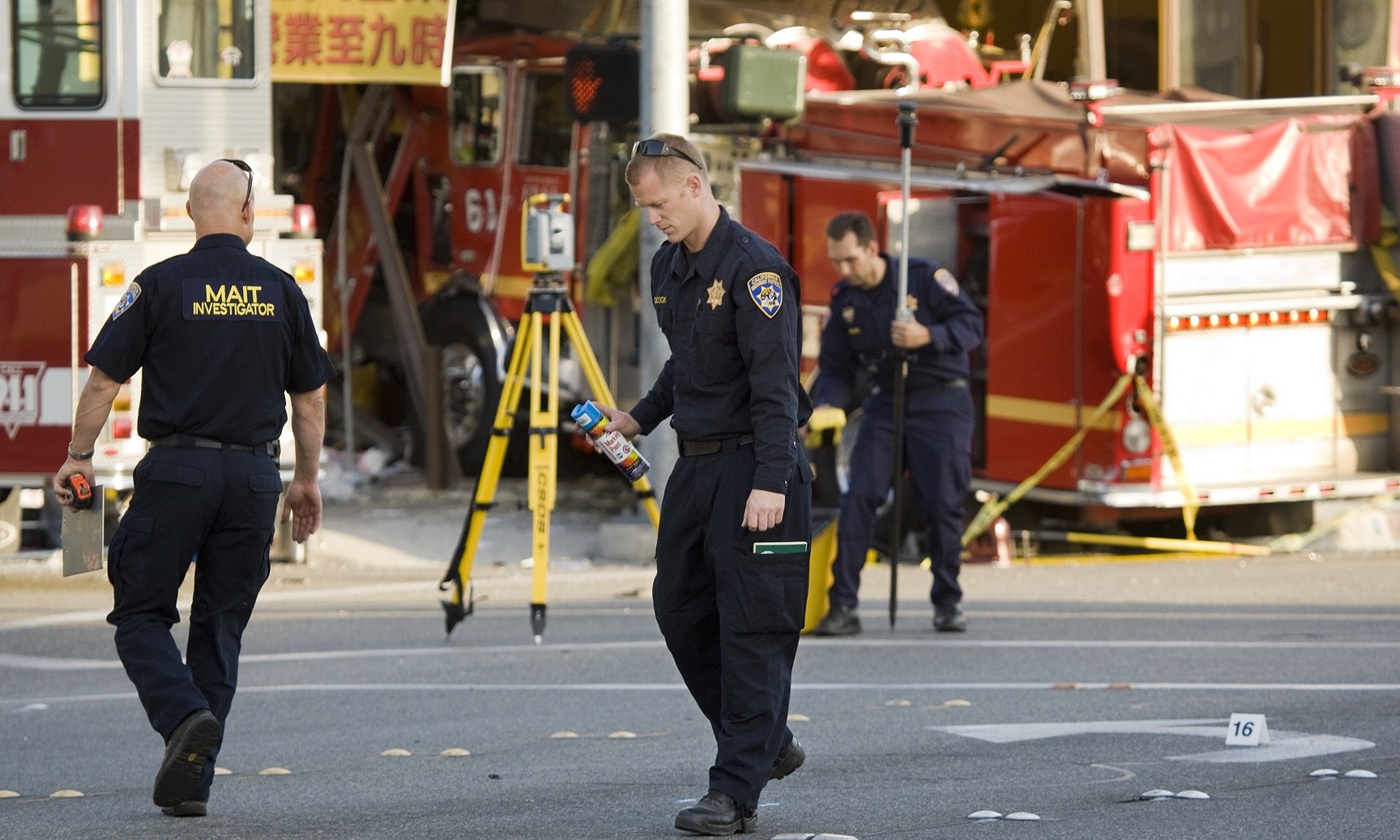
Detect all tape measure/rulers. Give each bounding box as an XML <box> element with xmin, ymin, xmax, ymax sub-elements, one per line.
<box><xmin>67</xmin><ymin>474</ymin><xmax>92</xmax><ymax>511</ymax></box>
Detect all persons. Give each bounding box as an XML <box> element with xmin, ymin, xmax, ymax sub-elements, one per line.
<box><xmin>806</xmin><ymin>212</ymin><xmax>984</xmax><ymax>637</ymax></box>
<box><xmin>54</xmin><ymin>157</ymin><xmax>339</xmax><ymax>817</ymax></box>
<box><xmin>573</xmin><ymin>133</ymin><xmax>816</xmax><ymax>835</ymax></box>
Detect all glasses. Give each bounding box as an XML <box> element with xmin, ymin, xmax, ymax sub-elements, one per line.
<box><xmin>631</xmin><ymin>140</ymin><xmax>703</xmax><ymax>169</ymax></box>
<box><xmin>217</xmin><ymin>159</ymin><xmax>253</xmax><ymax>212</ymax></box>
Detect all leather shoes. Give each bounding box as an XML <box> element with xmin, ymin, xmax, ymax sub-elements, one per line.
<box><xmin>153</xmin><ymin>709</ymin><xmax>222</xmax><ymax>807</ymax></box>
<box><xmin>161</xmin><ymin>803</ymin><xmax>206</xmax><ymax>817</ymax></box>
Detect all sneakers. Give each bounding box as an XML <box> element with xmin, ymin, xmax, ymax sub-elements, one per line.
<box><xmin>769</xmin><ymin>736</ymin><xmax>804</xmax><ymax>778</ymax></box>
<box><xmin>816</xmin><ymin>602</ymin><xmax>861</xmax><ymax>634</ymax></box>
<box><xmin>676</xmin><ymin>791</ymin><xmax>758</xmax><ymax>835</ymax></box>
<box><xmin>935</xmin><ymin>605</ymin><xmax>966</xmax><ymax>631</ymax></box>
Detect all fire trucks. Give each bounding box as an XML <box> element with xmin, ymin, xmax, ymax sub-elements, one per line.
<box><xmin>303</xmin><ymin>0</ymin><xmax>1398</xmax><ymax>522</ymax></box>
<box><xmin>1</xmin><ymin>0</ymin><xmax>330</xmax><ymax>544</ymax></box>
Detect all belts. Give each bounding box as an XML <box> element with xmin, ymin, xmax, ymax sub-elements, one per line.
<box><xmin>684</xmin><ymin>432</ymin><xmax>752</xmax><ymax>457</ymax></box>
<box><xmin>149</xmin><ymin>435</ymin><xmax>277</xmax><ymax>455</ymax></box>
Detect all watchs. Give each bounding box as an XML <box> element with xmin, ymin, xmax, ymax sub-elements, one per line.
<box><xmin>64</xmin><ymin>443</ymin><xmax>97</xmax><ymax>466</ymax></box>
